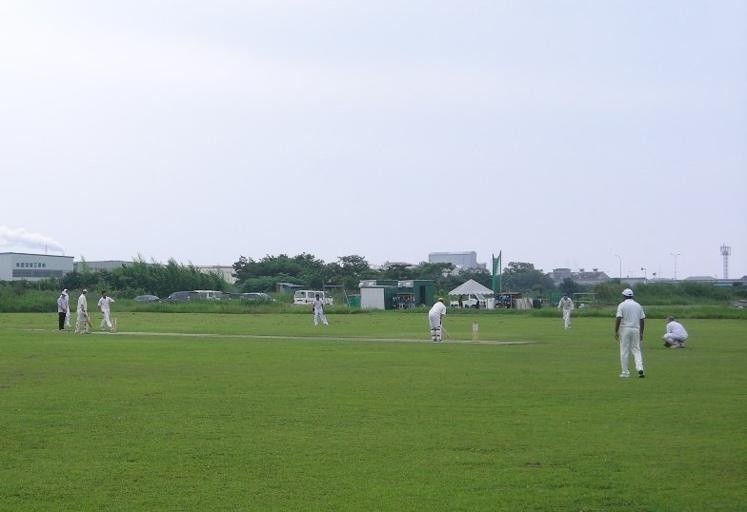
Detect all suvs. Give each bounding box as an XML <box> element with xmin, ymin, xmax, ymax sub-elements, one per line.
<box><xmin>193</xmin><ymin>290</ymin><xmax>279</xmax><ymax>305</ymax></box>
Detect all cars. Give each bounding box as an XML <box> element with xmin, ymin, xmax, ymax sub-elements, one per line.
<box><xmin>133</xmin><ymin>294</ymin><xmax>161</xmax><ymax>305</ymax></box>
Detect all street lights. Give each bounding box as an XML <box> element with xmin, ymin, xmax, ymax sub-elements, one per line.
<box><xmin>668</xmin><ymin>249</ymin><xmax>681</xmax><ymax>280</ymax></box>
<box><xmin>614</xmin><ymin>254</ymin><xmax>622</xmax><ymax>285</ymax></box>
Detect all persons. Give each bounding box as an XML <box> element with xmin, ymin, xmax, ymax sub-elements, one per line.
<box><xmin>73</xmin><ymin>288</ymin><xmax>91</xmax><ymax>335</ymax></box>
<box><xmin>94</xmin><ymin>289</ymin><xmax>116</xmax><ymax>330</ymax></box>
<box><xmin>556</xmin><ymin>291</ymin><xmax>575</xmax><ymax>330</ymax></box>
<box><xmin>427</xmin><ymin>297</ymin><xmax>447</xmax><ymax>344</ymax></box>
<box><xmin>57</xmin><ymin>288</ymin><xmax>71</xmax><ymax>327</ymax></box>
<box><xmin>612</xmin><ymin>286</ymin><xmax>647</xmax><ymax>380</ymax></box>
<box><xmin>56</xmin><ymin>291</ymin><xmax>67</xmax><ymax>330</ymax></box>
<box><xmin>311</xmin><ymin>293</ymin><xmax>330</xmax><ymax>327</ymax></box>
<box><xmin>661</xmin><ymin>314</ymin><xmax>690</xmax><ymax>349</ymax></box>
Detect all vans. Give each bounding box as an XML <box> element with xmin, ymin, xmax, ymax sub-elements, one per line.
<box><xmin>161</xmin><ymin>291</ymin><xmax>202</xmax><ymax>306</ymax></box>
<box><xmin>290</xmin><ymin>289</ymin><xmax>334</xmax><ymax>307</ymax></box>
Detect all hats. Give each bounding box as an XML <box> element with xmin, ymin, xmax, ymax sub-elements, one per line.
<box><xmin>60</xmin><ymin>288</ymin><xmax>107</xmax><ymax>297</ymax></box>
<box><xmin>622</xmin><ymin>288</ymin><xmax>633</xmax><ymax>297</ymax></box>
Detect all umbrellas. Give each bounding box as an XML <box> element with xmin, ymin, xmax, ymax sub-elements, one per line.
<box><xmin>447</xmin><ymin>278</ymin><xmax>495</xmax><ymax>309</ymax></box>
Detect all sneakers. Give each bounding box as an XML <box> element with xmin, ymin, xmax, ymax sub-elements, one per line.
<box><xmin>670</xmin><ymin>343</ymin><xmax>679</xmax><ymax>348</ymax></box>
<box><xmin>620</xmin><ymin>373</ymin><xmax>630</xmax><ymax>378</ymax></box>
<box><xmin>638</xmin><ymin>368</ymin><xmax>645</xmax><ymax>378</ymax></box>
<box><xmin>680</xmin><ymin>343</ymin><xmax>688</xmax><ymax>348</ymax></box>
